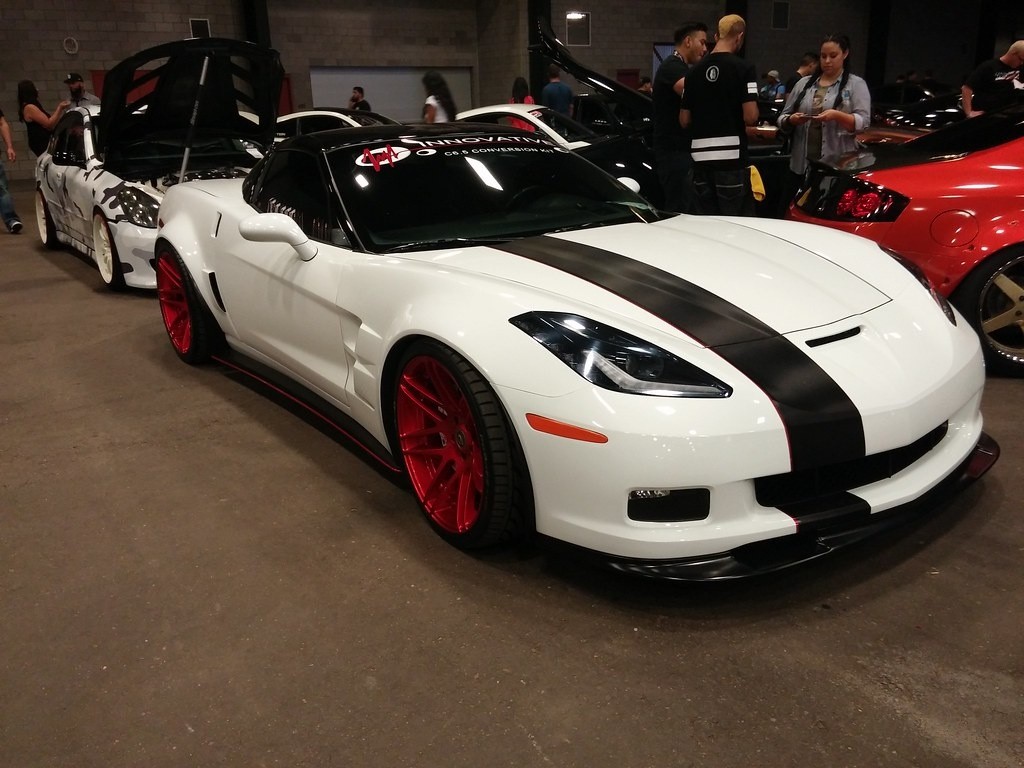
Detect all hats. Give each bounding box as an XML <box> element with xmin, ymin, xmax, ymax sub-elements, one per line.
<box><xmin>768</xmin><ymin>70</ymin><xmax>781</xmax><ymax>82</ymax></box>
<box><xmin>63</xmin><ymin>73</ymin><xmax>84</xmax><ymax>83</ymax></box>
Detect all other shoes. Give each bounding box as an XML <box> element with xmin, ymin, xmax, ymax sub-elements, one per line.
<box><xmin>7</xmin><ymin>219</ymin><xmax>23</xmax><ymax>234</ymax></box>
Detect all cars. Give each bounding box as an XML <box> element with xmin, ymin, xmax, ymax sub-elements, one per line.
<box><xmin>523</xmin><ymin>12</ymin><xmax>1024</xmax><ymax>220</ymax></box>
<box><xmin>784</xmin><ymin>114</ymin><xmax>1023</xmax><ymax>372</ymax></box>
<box><xmin>151</xmin><ymin>125</ymin><xmax>1004</xmax><ymax>607</ymax></box>
<box><xmin>270</xmin><ymin>98</ymin><xmax>609</xmax><ymax>166</ymax></box>
<box><xmin>34</xmin><ymin>32</ymin><xmax>297</xmax><ymax>295</ymax></box>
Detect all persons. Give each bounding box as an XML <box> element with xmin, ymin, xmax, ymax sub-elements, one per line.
<box><xmin>540</xmin><ymin>63</ymin><xmax>574</xmax><ymax>131</ymax></box>
<box><xmin>420</xmin><ymin>71</ymin><xmax>458</xmax><ymax>123</ymax></box>
<box><xmin>0</xmin><ymin>108</ymin><xmax>23</xmax><ymax>233</ymax></box>
<box><xmin>348</xmin><ymin>86</ymin><xmax>372</xmax><ymax>112</ymax></box>
<box><xmin>17</xmin><ymin>80</ymin><xmax>72</xmax><ymax>159</ymax></box>
<box><xmin>508</xmin><ymin>77</ymin><xmax>541</xmax><ymax>134</ymax></box>
<box><xmin>963</xmin><ymin>40</ymin><xmax>1024</xmax><ymax>119</ymax></box>
<box><xmin>59</xmin><ymin>73</ymin><xmax>101</xmax><ymax>112</ymax></box>
<box><xmin>640</xmin><ymin>14</ymin><xmax>934</xmax><ymax>216</ymax></box>
